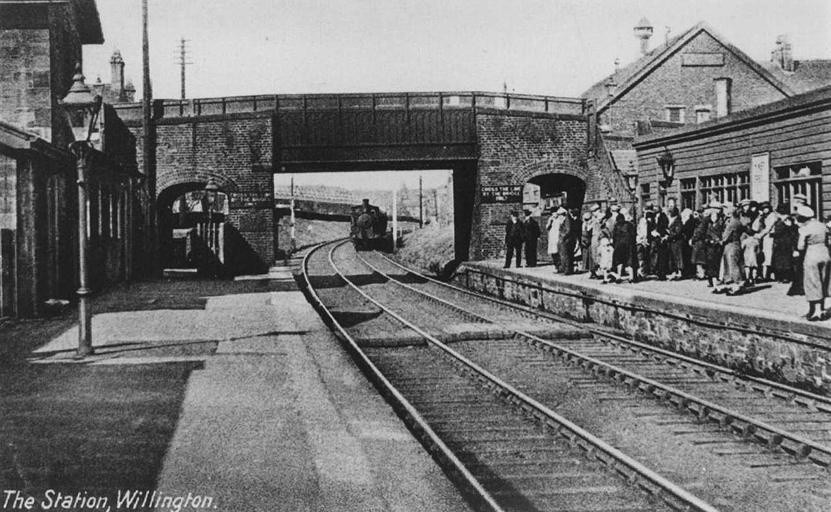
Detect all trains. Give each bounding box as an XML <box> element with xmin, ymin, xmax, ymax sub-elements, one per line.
<box><xmin>275</xmin><ymin>199</ymin><xmax>388</xmax><ymax>251</ymax></box>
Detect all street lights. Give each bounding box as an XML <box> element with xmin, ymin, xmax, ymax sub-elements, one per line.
<box><xmin>205</xmin><ymin>178</ymin><xmax>218</xmax><ymax>248</ymax></box>
<box><xmin>57</xmin><ymin>62</ymin><xmax>101</xmax><ymax>357</ymax></box>
<box><xmin>656</xmin><ymin>153</ymin><xmax>675</xmax><ymax>207</ymax></box>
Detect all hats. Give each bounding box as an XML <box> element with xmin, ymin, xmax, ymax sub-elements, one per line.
<box><xmin>751</xmin><ymin>201</ymin><xmax>758</xmax><ymax>209</ymax></box>
<box><xmin>591</xmin><ymin>204</ymin><xmax>599</xmax><ymax>210</ymax></box>
<box><xmin>550</xmin><ymin>207</ymin><xmax>557</xmax><ymax>213</ymax></box>
<box><xmin>797</xmin><ymin>206</ymin><xmax>815</xmax><ymax>217</ymax></box>
<box><xmin>611</xmin><ymin>206</ymin><xmax>621</xmax><ymax>210</ymax></box>
<box><xmin>512</xmin><ymin>212</ymin><xmax>517</xmax><ymax>217</ymax></box>
<box><xmin>759</xmin><ymin>201</ymin><xmax>772</xmax><ymax>209</ymax></box>
<box><xmin>794</xmin><ymin>193</ymin><xmax>807</xmax><ymax>201</ymax></box>
<box><xmin>724</xmin><ymin>206</ymin><xmax>736</xmax><ymax>216</ymax></box>
<box><xmin>584</xmin><ymin>212</ymin><xmax>591</xmax><ymax>219</ymax></box>
<box><xmin>525</xmin><ymin>209</ymin><xmax>532</xmax><ymax>216</ymax></box>
<box><xmin>742</xmin><ymin>200</ymin><xmax>751</xmax><ymax>206</ymax></box>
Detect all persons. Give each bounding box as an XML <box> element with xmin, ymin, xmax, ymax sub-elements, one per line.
<box><xmin>505</xmin><ymin>196</ymin><xmax>831</xmax><ymax>324</ymax></box>
